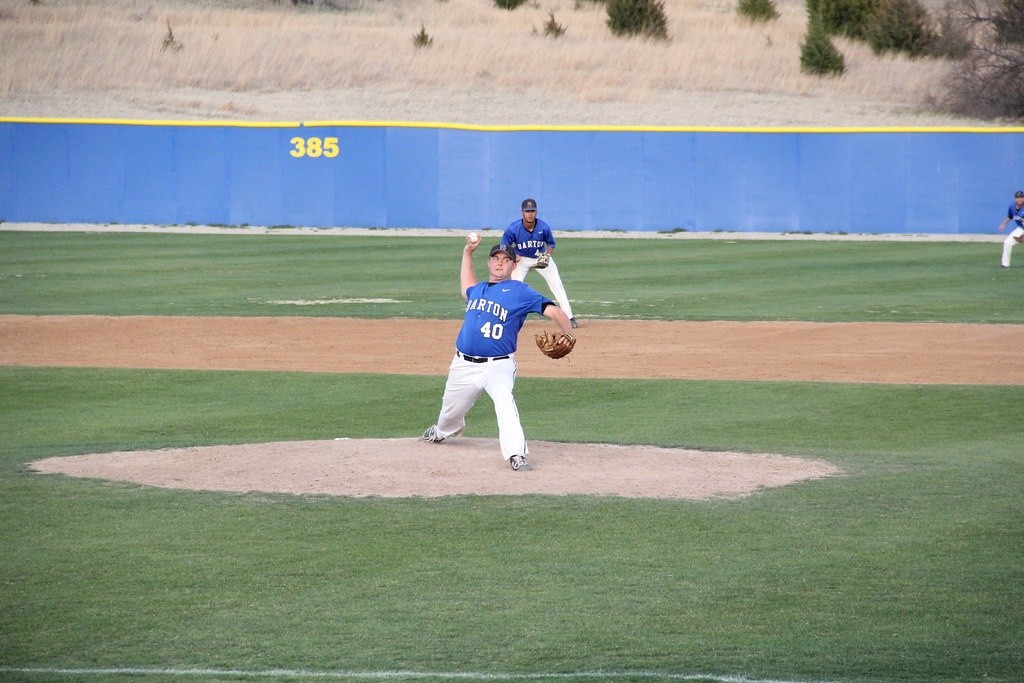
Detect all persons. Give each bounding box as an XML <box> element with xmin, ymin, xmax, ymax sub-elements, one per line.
<box><xmin>999</xmin><ymin>191</ymin><xmax>1024</xmax><ymax>268</ymax></box>
<box><xmin>501</xmin><ymin>199</ymin><xmax>578</xmax><ymax>328</ymax></box>
<box><xmin>422</xmin><ymin>232</ymin><xmax>577</xmax><ymax>472</ymax></box>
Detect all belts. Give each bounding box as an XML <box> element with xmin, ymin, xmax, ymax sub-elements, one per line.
<box><xmin>457</xmin><ymin>350</ymin><xmax>509</xmax><ymax>363</ymax></box>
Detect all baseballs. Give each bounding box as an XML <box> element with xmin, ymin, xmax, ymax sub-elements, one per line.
<box><xmin>469</xmin><ymin>232</ymin><xmax>478</xmax><ymax>244</ymax></box>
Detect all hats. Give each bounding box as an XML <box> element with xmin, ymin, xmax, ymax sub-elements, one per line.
<box><xmin>489</xmin><ymin>243</ymin><xmax>516</xmax><ymax>262</ymax></box>
<box><xmin>522</xmin><ymin>199</ymin><xmax>537</xmax><ymax>210</ymax></box>
<box><xmin>1015</xmin><ymin>191</ymin><xmax>1024</xmax><ymax>197</ymax></box>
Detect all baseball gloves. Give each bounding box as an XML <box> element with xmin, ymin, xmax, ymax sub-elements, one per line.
<box><xmin>529</xmin><ymin>253</ymin><xmax>550</xmax><ymax>272</ymax></box>
<box><xmin>533</xmin><ymin>329</ymin><xmax>577</xmax><ymax>363</ymax></box>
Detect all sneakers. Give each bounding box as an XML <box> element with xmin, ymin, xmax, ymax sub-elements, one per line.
<box><xmin>421</xmin><ymin>425</ymin><xmax>445</xmax><ymax>442</ymax></box>
<box><xmin>510</xmin><ymin>455</ymin><xmax>532</xmax><ymax>472</ymax></box>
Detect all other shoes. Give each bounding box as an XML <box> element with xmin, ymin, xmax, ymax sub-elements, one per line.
<box><xmin>570</xmin><ymin>318</ymin><xmax>577</xmax><ymax>328</ymax></box>
